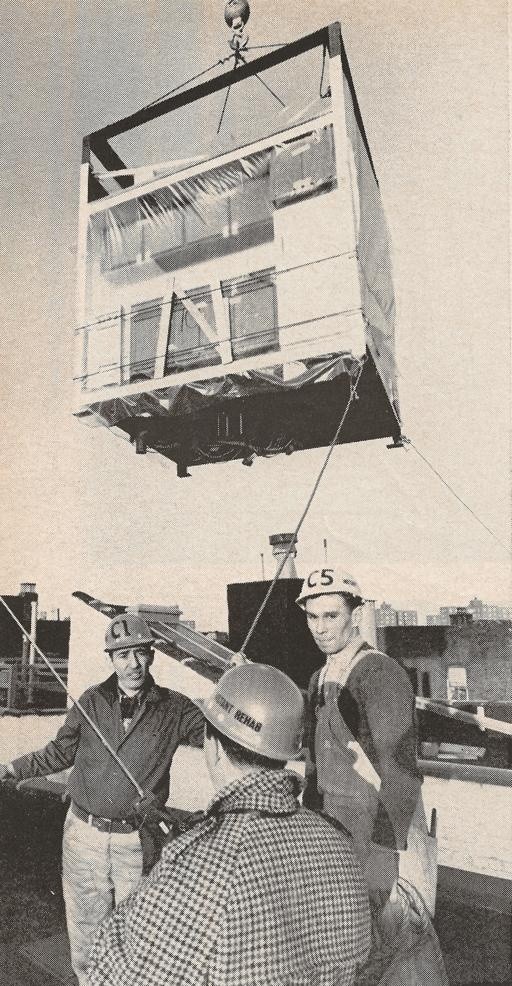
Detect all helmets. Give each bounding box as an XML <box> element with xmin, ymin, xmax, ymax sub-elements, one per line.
<box><xmin>295</xmin><ymin>568</ymin><xmax>367</xmax><ymax>612</ymax></box>
<box><xmin>105</xmin><ymin>613</ymin><xmax>155</xmax><ymax>652</ymax></box>
<box><xmin>198</xmin><ymin>663</ymin><xmax>306</xmax><ymax>763</ymax></box>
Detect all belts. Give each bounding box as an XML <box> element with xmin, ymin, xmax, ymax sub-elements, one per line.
<box><xmin>71</xmin><ymin>802</ymin><xmax>142</xmax><ymax>834</ymax></box>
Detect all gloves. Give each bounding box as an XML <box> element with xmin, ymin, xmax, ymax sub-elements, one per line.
<box><xmin>363</xmin><ymin>841</ymin><xmax>398</xmax><ymax>907</ymax></box>
<box><xmin>0</xmin><ymin>762</ymin><xmax>19</xmax><ymax>788</ymax></box>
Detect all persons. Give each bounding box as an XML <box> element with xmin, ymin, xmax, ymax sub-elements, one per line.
<box><xmin>295</xmin><ymin>565</ymin><xmax>447</xmax><ymax>985</ymax></box>
<box><xmin>1</xmin><ymin>612</ymin><xmax>204</xmax><ymax>986</ymax></box>
<box><xmin>80</xmin><ymin>662</ymin><xmax>373</xmax><ymax>986</ymax></box>
